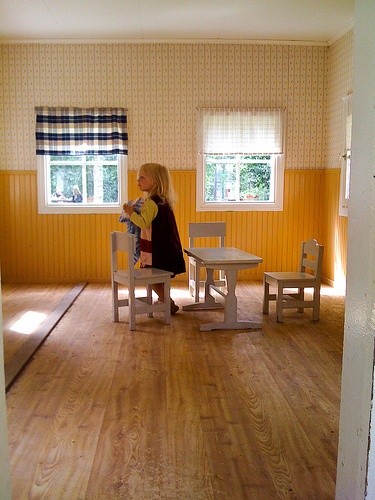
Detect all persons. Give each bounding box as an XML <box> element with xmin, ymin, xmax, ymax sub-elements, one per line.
<box><xmin>122</xmin><ymin>163</ymin><xmax>186</xmax><ymax>319</ymax></box>
<box><xmin>69</xmin><ymin>185</ymin><xmax>83</xmax><ymax>203</ymax></box>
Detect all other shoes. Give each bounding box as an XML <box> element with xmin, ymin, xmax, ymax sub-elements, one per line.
<box><xmin>154</xmin><ymin>299</ymin><xmax>179</xmax><ymax>314</ymax></box>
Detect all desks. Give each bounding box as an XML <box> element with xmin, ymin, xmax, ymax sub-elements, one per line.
<box><xmin>182</xmin><ymin>246</ymin><xmax>263</xmax><ymax>332</ymax></box>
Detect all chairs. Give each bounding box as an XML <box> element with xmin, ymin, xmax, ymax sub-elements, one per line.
<box><xmin>261</xmin><ymin>237</ymin><xmax>324</xmax><ymax>323</ymax></box>
<box><xmin>110</xmin><ymin>231</ymin><xmax>174</xmax><ymax>332</ymax></box>
<box><xmin>188</xmin><ymin>222</ymin><xmax>226</xmax><ymax>302</ymax></box>
